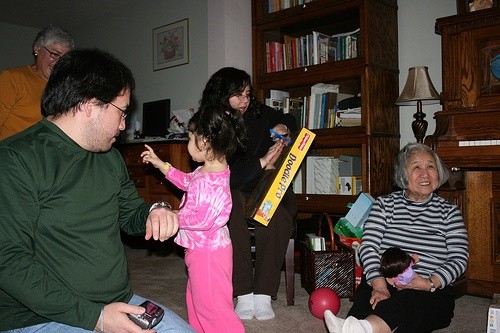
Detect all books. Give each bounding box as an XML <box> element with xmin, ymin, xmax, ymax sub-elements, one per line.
<box><xmin>265</xmin><ymin>83</ymin><xmax>362</xmax><ymax>130</ymax></box>
<box><xmin>262</xmin><ymin>0</ymin><xmax>314</xmax><ymax>13</ymax></box>
<box><xmin>265</xmin><ymin>28</ymin><xmax>362</xmax><ymax>73</ymax></box>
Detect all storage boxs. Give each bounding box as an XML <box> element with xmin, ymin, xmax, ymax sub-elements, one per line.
<box><xmin>314</xmin><ymin>159</ymin><xmax>338</xmax><ymax>194</ymax></box>
<box><xmin>487</xmin><ymin>294</ymin><xmax>500</xmax><ymax>333</ymax></box>
<box><xmin>334</xmin><ymin>193</ymin><xmax>375</xmax><ymax>239</ymax></box>
<box><xmin>245</xmin><ymin>127</ymin><xmax>316</xmax><ymax>227</ymax></box>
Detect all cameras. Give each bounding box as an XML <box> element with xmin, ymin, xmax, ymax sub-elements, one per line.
<box><xmin>127</xmin><ymin>300</ymin><xmax>164</xmax><ymax>330</ymax></box>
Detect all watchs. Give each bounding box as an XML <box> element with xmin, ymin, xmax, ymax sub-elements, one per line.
<box><xmin>149</xmin><ymin>199</ymin><xmax>173</xmax><ymax>213</ymax></box>
<box><xmin>428</xmin><ymin>278</ymin><xmax>436</xmax><ymax>293</ymax></box>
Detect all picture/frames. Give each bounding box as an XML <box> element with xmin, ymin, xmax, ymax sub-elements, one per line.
<box><xmin>152</xmin><ymin>18</ymin><xmax>189</xmax><ymax>71</ymax></box>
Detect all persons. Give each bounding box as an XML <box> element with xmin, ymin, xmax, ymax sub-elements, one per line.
<box><xmin>140</xmin><ymin>104</ymin><xmax>249</xmax><ymax>333</ymax></box>
<box><xmin>188</xmin><ymin>66</ymin><xmax>296</xmax><ymax>322</ymax></box>
<box><xmin>0</xmin><ymin>48</ymin><xmax>179</xmax><ymax>333</ymax></box>
<box><xmin>380</xmin><ymin>247</ymin><xmax>419</xmax><ymax>291</ymax></box>
<box><xmin>323</xmin><ymin>142</ymin><xmax>470</xmax><ymax>333</ymax></box>
<box><xmin>0</xmin><ymin>26</ymin><xmax>78</xmax><ymax>143</ymax></box>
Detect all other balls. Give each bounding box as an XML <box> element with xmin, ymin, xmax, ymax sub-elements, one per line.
<box><xmin>308</xmin><ymin>287</ymin><xmax>341</xmax><ymax>320</ymax></box>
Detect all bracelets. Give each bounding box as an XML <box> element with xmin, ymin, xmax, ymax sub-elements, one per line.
<box><xmin>100</xmin><ymin>305</ymin><xmax>104</xmax><ymax>333</ymax></box>
<box><xmin>160</xmin><ymin>161</ymin><xmax>172</xmax><ymax>175</ymax></box>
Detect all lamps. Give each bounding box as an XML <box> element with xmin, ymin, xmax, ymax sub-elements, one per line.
<box><xmin>395</xmin><ymin>65</ymin><xmax>441</xmax><ymax>144</ymax></box>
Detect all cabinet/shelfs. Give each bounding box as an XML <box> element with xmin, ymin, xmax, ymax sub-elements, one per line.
<box><xmin>424</xmin><ymin>1</ymin><xmax>500</xmax><ymax>299</ymax></box>
<box><xmin>113</xmin><ymin>140</ymin><xmax>192</xmax><ymax>209</ymax></box>
<box><xmin>251</xmin><ymin>0</ymin><xmax>401</xmax><ymax>274</ymax></box>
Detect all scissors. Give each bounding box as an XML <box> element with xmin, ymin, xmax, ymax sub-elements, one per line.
<box><xmin>269</xmin><ymin>129</ymin><xmax>292</xmax><ymax>143</ymax></box>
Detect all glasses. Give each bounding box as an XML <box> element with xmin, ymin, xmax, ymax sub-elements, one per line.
<box><xmin>44</xmin><ymin>46</ymin><xmax>63</xmax><ymax>62</ymax></box>
<box><xmin>235</xmin><ymin>94</ymin><xmax>254</xmax><ymax>102</ymax></box>
<box><xmin>108</xmin><ymin>102</ymin><xmax>129</xmax><ymax>119</ymax></box>
